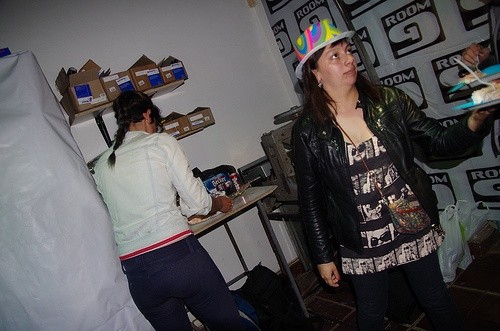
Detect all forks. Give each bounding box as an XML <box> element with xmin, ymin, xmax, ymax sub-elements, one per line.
<box><xmin>454</xmin><ymin>57</ymin><xmax>497</xmax><ymax>91</ymax></box>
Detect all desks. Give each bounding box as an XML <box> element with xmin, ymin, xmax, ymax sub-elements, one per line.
<box><xmin>186</xmin><ymin>186</ymin><xmax>311</xmax><ymax>331</ymax></box>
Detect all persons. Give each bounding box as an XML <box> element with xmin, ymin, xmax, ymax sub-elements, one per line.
<box><xmin>292</xmin><ymin>19</ymin><xmax>500</xmax><ymax>331</ymax></box>
<box><xmin>95</xmin><ymin>90</ymin><xmax>249</xmax><ymax>331</ymax></box>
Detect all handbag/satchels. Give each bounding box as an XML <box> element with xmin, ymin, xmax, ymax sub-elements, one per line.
<box><xmin>437</xmin><ymin>199</ymin><xmax>490</xmax><ymax>284</ymax></box>
<box><xmin>231</xmin><ymin>265</ymin><xmax>315</xmax><ymax>331</ymax></box>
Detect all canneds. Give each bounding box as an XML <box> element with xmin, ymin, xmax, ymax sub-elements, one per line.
<box><xmin>240</xmin><ymin>193</ymin><xmax>249</xmax><ymax>205</ymax></box>
<box><xmin>229</xmin><ymin>173</ymin><xmax>243</xmax><ymax>191</ymax></box>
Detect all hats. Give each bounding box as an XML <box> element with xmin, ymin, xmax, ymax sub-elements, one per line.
<box><xmin>295</xmin><ymin>19</ymin><xmax>355</xmax><ymax>80</ymax></box>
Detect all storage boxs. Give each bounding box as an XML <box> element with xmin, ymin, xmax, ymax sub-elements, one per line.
<box><xmin>203</xmin><ymin>173</ymin><xmax>243</xmax><ymax>196</ymax></box>
<box><xmin>161</xmin><ymin>107</ymin><xmax>215</xmax><ymax>137</ymax></box>
<box><xmin>54</xmin><ymin>54</ymin><xmax>189</xmax><ymax>116</ymax></box>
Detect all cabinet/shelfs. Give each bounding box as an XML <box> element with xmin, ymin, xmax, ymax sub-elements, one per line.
<box><xmin>69</xmin><ymin>79</ymin><xmax>204</xmax><ymax>173</ymax></box>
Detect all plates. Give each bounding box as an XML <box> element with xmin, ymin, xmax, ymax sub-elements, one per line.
<box><xmin>186</xmin><ymin>194</ymin><xmax>229</xmax><ymax>226</ymax></box>
<box><xmin>447</xmin><ymin>64</ymin><xmax>500</xmax><ymax>93</ymax></box>
<box><xmin>452</xmin><ymin>97</ymin><xmax>500</xmax><ymax>111</ymax></box>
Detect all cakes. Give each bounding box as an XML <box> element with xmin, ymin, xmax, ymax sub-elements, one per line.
<box><xmin>459</xmin><ymin>71</ymin><xmax>486</xmax><ymax>83</ymax></box>
<box><xmin>471</xmin><ymin>84</ymin><xmax>500</xmax><ymax>103</ymax></box>
